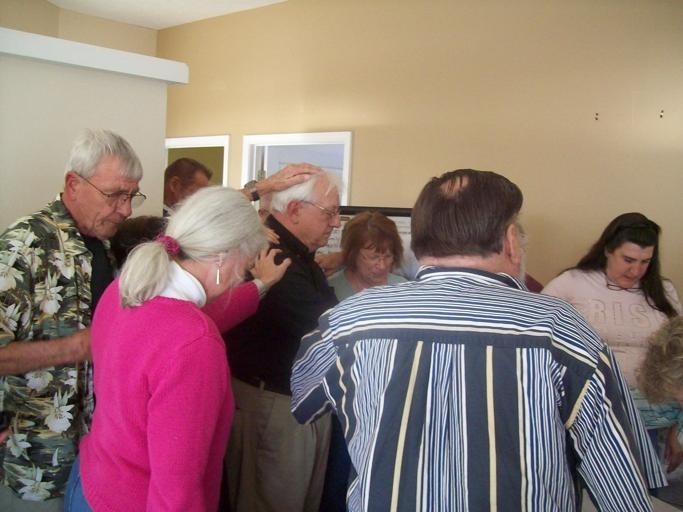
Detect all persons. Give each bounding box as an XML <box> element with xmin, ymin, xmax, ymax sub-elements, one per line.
<box><xmin>0</xmin><ymin>127</ymin><xmax>682</xmax><ymax>512</ymax></box>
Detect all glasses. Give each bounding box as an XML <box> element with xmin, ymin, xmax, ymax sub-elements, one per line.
<box><xmin>299</xmin><ymin>200</ymin><xmax>342</xmax><ymax>216</ymax></box>
<box><xmin>514</xmin><ymin>221</ymin><xmax>529</xmax><ymax>246</ymax></box>
<box><xmin>74</xmin><ymin>171</ymin><xmax>146</xmax><ymax>208</ymax></box>
<box><xmin>604</xmin><ymin>271</ymin><xmax>644</xmax><ymax>294</ymax></box>
<box><xmin>358</xmin><ymin>251</ymin><xmax>394</xmax><ymax>264</ymax></box>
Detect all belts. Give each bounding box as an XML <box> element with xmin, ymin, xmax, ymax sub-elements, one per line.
<box><xmin>230</xmin><ymin>371</ymin><xmax>292</xmax><ymax>395</ymax></box>
